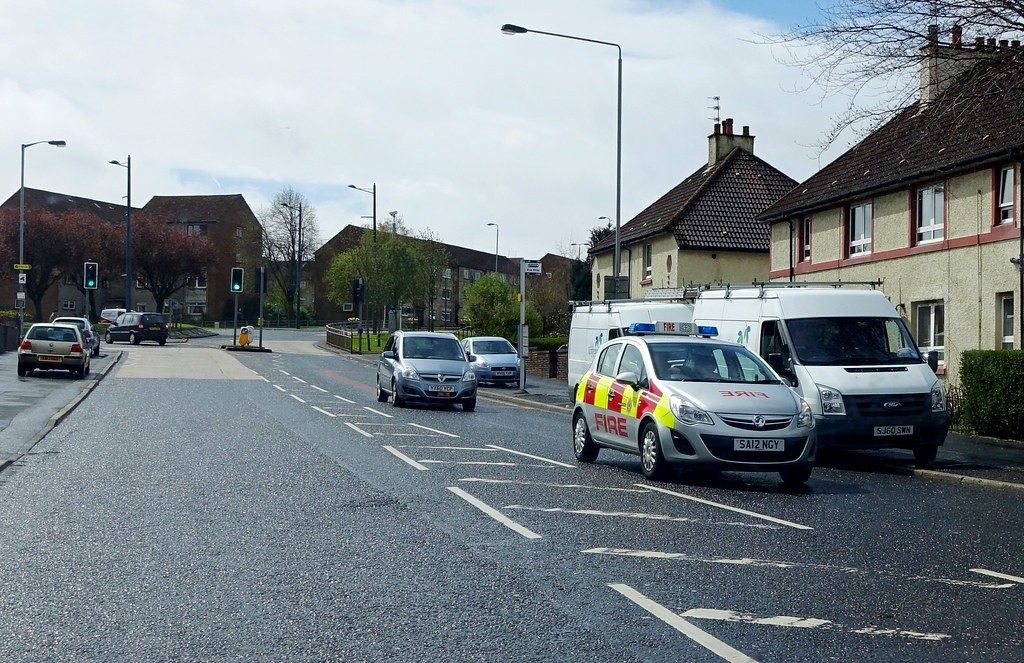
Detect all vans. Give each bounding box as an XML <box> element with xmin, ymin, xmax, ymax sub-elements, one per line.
<box><xmin>689</xmin><ymin>279</ymin><xmax>951</xmax><ymax>461</ymax></box>
<box><xmin>567</xmin><ymin>285</ymin><xmax>705</xmax><ymax>408</ymax></box>
<box><xmin>100</xmin><ymin>309</ymin><xmax>138</xmax><ymax>324</ymax></box>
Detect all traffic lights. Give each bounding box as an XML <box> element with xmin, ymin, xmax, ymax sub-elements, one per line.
<box><xmin>230</xmin><ymin>267</ymin><xmax>243</xmax><ymax>292</ymax></box>
<box><xmin>83</xmin><ymin>262</ymin><xmax>97</xmax><ymax>289</ymax></box>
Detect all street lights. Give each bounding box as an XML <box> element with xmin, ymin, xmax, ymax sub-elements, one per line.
<box><xmin>17</xmin><ymin>140</ymin><xmax>65</xmax><ymax>344</ymax></box>
<box><xmin>109</xmin><ymin>154</ymin><xmax>132</xmax><ymax>313</ymax></box>
<box><xmin>348</xmin><ymin>183</ymin><xmax>378</xmax><ymax>335</ymax></box>
<box><xmin>281</xmin><ymin>202</ymin><xmax>302</xmax><ymax>329</ymax></box>
<box><xmin>500</xmin><ymin>23</ymin><xmax>622</xmax><ymax>278</ymax></box>
<box><xmin>486</xmin><ymin>223</ymin><xmax>499</xmax><ymax>273</ymax></box>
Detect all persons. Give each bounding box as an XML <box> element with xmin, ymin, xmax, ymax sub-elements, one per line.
<box><xmin>49</xmin><ymin>310</ymin><xmax>59</xmax><ymax>323</ymax></box>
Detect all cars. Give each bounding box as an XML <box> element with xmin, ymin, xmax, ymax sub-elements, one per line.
<box><xmin>18</xmin><ymin>323</ymin><xmax>92</xmax><ymax>380</ymax></box>
<box><xmin>461</xmin><ymin>336</ymin><xmax>526</xmax><ymax>386</ymax></box>
<box><xmin>46</xmin><ymin>316</ymin><xmax>101</xmax><ymax>358</ymax></box>
<box><xmin>570</xmin><ymin>322</ymin><xmax>817</xmax><ymax>483</ymax></box>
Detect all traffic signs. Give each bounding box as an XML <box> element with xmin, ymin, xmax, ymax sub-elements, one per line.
<box><xmin>13</xmin><ymin>263</ymin><xmax>32</xmax><ymax>269</ymax></box>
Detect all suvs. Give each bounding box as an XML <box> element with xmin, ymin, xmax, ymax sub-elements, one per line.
<box><xmin>105</xmin><ymin>312</ymin><xmax>170</xmax><ymax>346</ymax></box>
<box><xmin>376</xmin><ymin>329</ymin><xmax>479</xmax><ymax>410</ymax></box>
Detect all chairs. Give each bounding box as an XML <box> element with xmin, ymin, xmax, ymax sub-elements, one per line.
<box><xmin>694</xmin><ymin>358</ymin><xmax>721</xmax><ymax>379</ymax></box>
<box><xmin>36</xmin><ymin>331</ymin><xmax>48</xmax><ymax>338</ymax></box>
<box><xmin>64</xmin><ymin>333</ymin><xmax>74</xmax><ymax>341</ymax></box>
